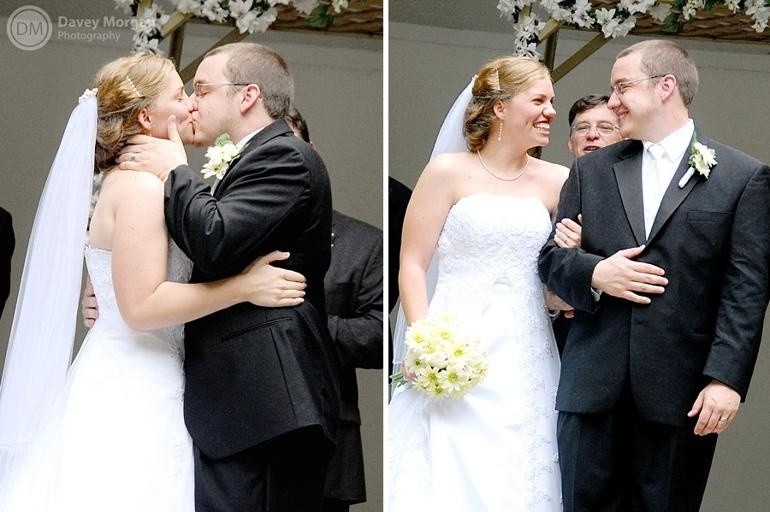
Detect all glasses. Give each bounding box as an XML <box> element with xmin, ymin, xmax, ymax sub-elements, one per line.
<box><xmin>193</xmin><ymin>82</ymin><xmax>249</xmax><ymax>96</ymax></box>
<box><xmin>571</xmin><ymin>120</ymin><xmax>621</xmax><ymax>136</ymax></box>
<box><xmin>607</xmin><ymin>74</ymin><xmax>661</xmax><ymax>96</ymax></box>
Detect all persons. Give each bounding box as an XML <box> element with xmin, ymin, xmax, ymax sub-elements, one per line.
<box><xmin>546</xmin><ymin>89</ymin><xmax>625</xmax><ymax>357</ymax></box>
<box><xmin>537</xmin><ymin>36</ymin><xmax>768</xmax><ymax>512</ymax></box>
<box><xmin>389</xmin><ymin>174</ymin><xmax>412</xmax><ymax>386</ymax></box>
<box><xmin>80</xmin><ymin>43</ymin><xmax>346</xmax><ymax>512</ymax></box>
<box><xmin>387</xmin><ymin>56</ymin><xmax>583</xmax><ymax>512</ymax></box>
<box><xmin>280</xmin><ymin>103</ymin><xmax>381</xmax><ymax>512</ymax></box>
<box><xmin>0</xmin><ymin>50</ymin><xmax>307</xmax><ymax>512</ymax></box>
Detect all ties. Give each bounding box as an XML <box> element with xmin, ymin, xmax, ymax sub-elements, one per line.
<box><xmin>644</xmin><ymin>142</ymin><xmax>669</xmax><ymax>241</ymax></box>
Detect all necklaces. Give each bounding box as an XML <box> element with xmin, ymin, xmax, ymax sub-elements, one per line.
<box><xmin>477</xmin><ymin>147</ymin><xmax>530</xmax><ymax>182</ymax></box>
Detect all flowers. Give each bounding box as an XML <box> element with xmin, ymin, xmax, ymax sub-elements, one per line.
<box><xmin>198</xmin><ymin>132</ymin><xmax>240</xmax><ymax>182</ymax></box>
<box><xmin>687</xmin><ymin>132</ymin><xmax>719</xmax><ymax>180</ymax></box>
<box><xmin>388</xmin><ymin>314</ymin><xmax>486</xmax><ymax>398</ymax></box>
<box><xmin>114</xmin><ymin>0</ymin><xmax>351</xmax><ymax>56</ymax></box>
<box><xmin>496</xmin><ymin>0</ymin><xmax>770</xmax><ymax>62</ymax></box>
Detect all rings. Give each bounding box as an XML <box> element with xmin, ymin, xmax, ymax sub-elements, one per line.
<box><xmin>131</xmin><ymin>152</ymin><xmax>137</xmax><ymax>162</ymax></box>
<box><xmin>564</xmin><ymin>236</ymin><xmax>571</xmax><ymax>243</ymax></box>
<box><xmin>719</xmin><ymin>416</ymin><xmax>728</xmax><ymax>422</ymax></box>
